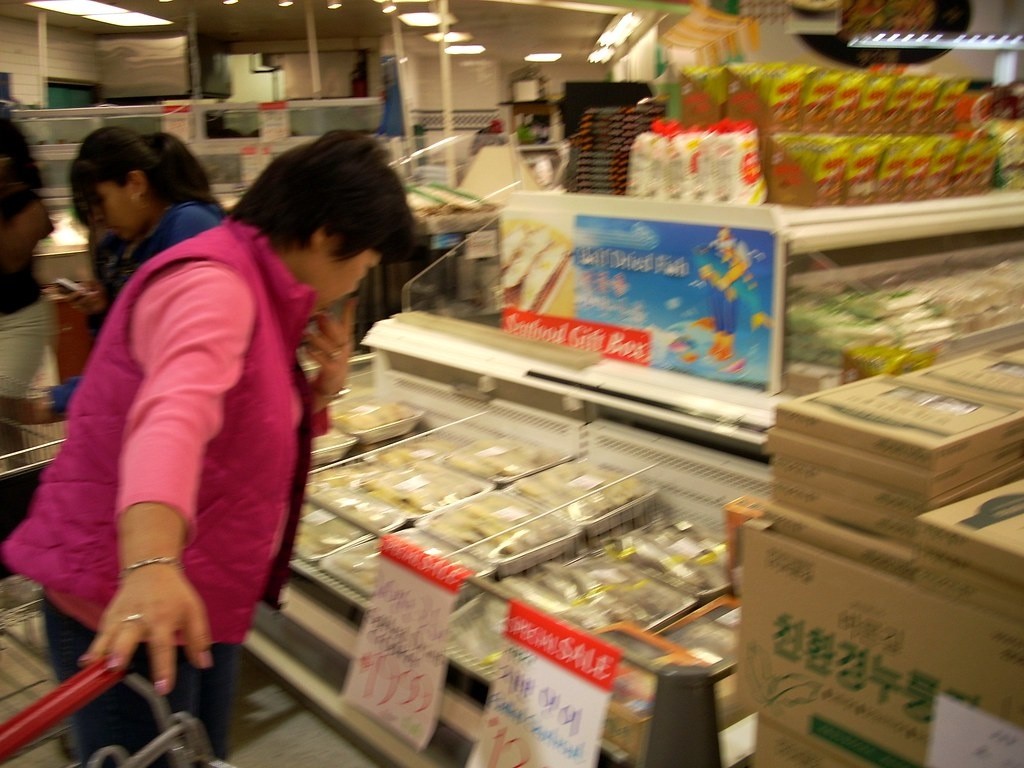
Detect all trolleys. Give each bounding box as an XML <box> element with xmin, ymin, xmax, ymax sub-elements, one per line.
<box><xmin>0</xmin><ymin>372</ymin><xmax>71</xmax><ymax>484</ymax></box>
<box><xmin>0</xmin><ymin>571</ymin><xmax>244</xmax><ymax>768</ymax></box>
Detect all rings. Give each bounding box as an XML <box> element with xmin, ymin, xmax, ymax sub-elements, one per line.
<box><xmin>122</xmin><ymin>615</ymin><xmax>142</xmax><ymax>622</ymax></box>
<box><xmin>329</xmin><ymin>350</ymin><xmax>342</xmax><ymax>357</ymax></box>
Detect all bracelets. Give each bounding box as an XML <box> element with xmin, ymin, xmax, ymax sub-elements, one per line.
<box><xmin>119</xmin><ymin>556</ymin><xmax>185</xmax><ymax>574</ymax></box>
<box><xmin>316</xmin><ymin>386</ymin><xmax>350</xmax><ymax>398</ymax></box>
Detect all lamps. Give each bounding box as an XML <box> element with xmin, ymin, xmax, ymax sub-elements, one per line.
<box><xmin>327</xmin><ymin>0</ymin><xmax>344</xmax><ymax>10</ymax></box>
<box><xmin>223</xmin><ymin>0</ymin><xmax>238</xmax><ymax>5</ymax></box>
<box><xmin>278</xmin><ymin>0</ymin><xmax>294</xmax><ymax>7</ymax></box>
<box><xmin>381</xmin><ymin>0</ymin><xmax>398</xmax><ymax>14</ymax></box>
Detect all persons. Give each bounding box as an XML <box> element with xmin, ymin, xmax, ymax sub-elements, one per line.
<box><xmin>0</xmin><ymin>127</ymin><xmax>418</xmax><ymax>768</ymax></box>
<box><xmin>0</xmin><ymin>109</ymin><xmax>58</xmax><ymax>429</ymax></box>
<box><xmin>49</xmin><ymin>126</ymin><xmax>227</xmax><ymax>425</ymax></box>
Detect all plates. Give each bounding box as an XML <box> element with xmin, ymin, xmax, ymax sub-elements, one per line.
<box><xmin>292</xmin><ymin>395</ymin><xmax>731</xmax><ymax>686</ymax></box>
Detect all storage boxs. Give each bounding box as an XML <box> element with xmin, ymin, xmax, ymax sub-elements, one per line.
<box><xmin>601</xmin><ymin>337</ymin><xmax>1024</xmax><ymax>768</ymax></box>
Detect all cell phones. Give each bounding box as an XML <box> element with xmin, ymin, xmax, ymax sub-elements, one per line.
<box><xmin>54</xmin><ymin>279</ymin><xmax>84</xmax><ymax>296</ymax></box>
